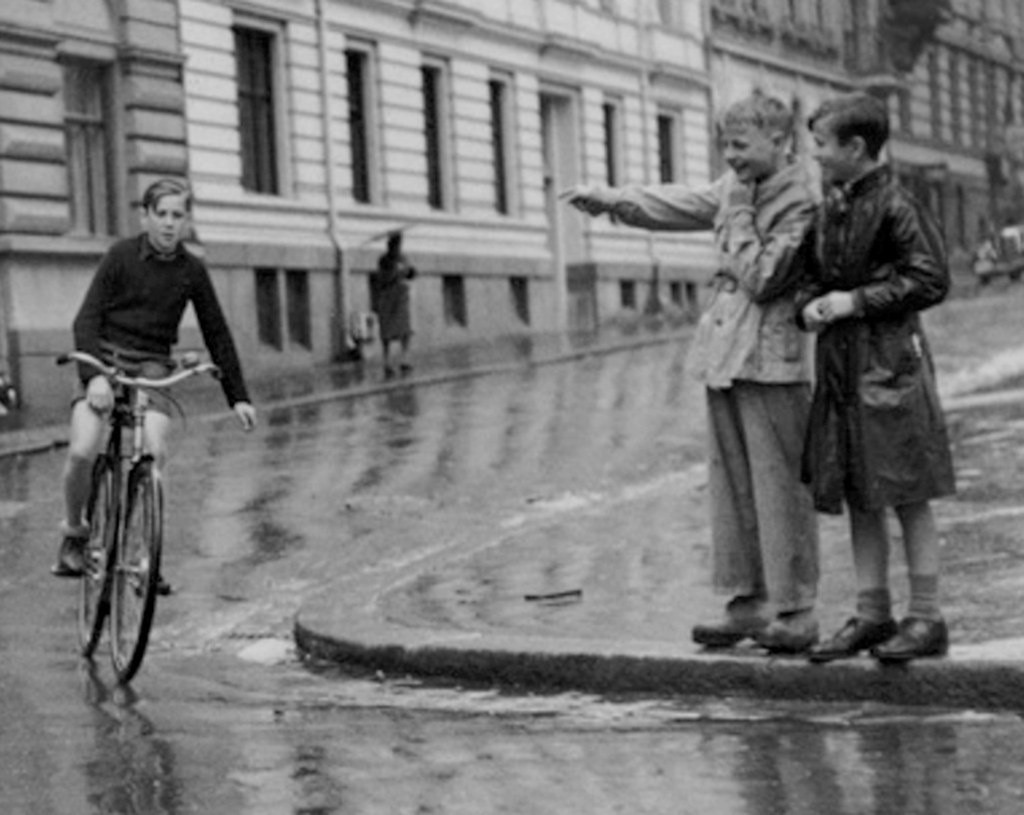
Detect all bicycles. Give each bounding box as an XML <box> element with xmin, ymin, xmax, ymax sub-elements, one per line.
<box><xmin>54</xmin><ymin>351</ymin><xmax>226</xmax><ymax>680</ymax></box>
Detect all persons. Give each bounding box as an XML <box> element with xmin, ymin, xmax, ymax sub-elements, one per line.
<box><xmin>372</xmin><ymin>229</ymin><xmax>416</xmax><ymax>380</ymax></box>
<box><xmin>52</xmin><ymin>178</ymin><xmax>255</xmax><ymax>596</ymax></box>
<box><xmin>558</xmin><ymin>95</ymin><xmax>816</xmax><ymax>651</ymax></box>
<box><xmin>792</xmin><ymin>93</ymin><xmax>952</xmax><ymax>668</ymax></box>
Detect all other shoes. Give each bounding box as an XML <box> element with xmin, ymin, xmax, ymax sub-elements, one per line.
<box><xmin>692</xmin><ymin>606</ymin><xmax>772</xmax><ymax>646</ymax></box>
<box><xmin>400</xmin><ymin>365</ymin><xmax>413</xmax><ymax>372</ymax></box>
<box><xmin>385</xmin><ymin>367</ymin><xmax>396</xmax><ymax>380</ymax></box>
<box><xmin>760</xmin><ymin>612</ymin><xmax>820</xmax><ymax>650</ymax></box>
<box><xmin>58</xmin><ymin>535</ymin><xmax>90</xmax><ymax>575</ymax></box>
<box><xmin>141</xmin><ymin>570</ymin><xmax>171</xmax><ymax>597</ymax></box>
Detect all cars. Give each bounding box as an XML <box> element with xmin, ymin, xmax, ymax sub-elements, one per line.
<box><xmin>974</xmin><ymin>225</ymin><xmax>1024</xmax><ymax>284</ymax></box>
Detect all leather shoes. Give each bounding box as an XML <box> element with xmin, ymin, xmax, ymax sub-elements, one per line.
<box><xmin>876</xmin><ymin>619</ymin><xmax>949</xmax><ymax>659</ymax></box>
<box><xmin>811</xmin><ymin>618</ymin><xmax>900</xmax><ymax>662</ymax></box>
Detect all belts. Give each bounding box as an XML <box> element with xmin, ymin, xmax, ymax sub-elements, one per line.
<box><xmin>97</xmin><ymin>342</ymin><xmax>164</xmax><ymax>364</ymax></box>
